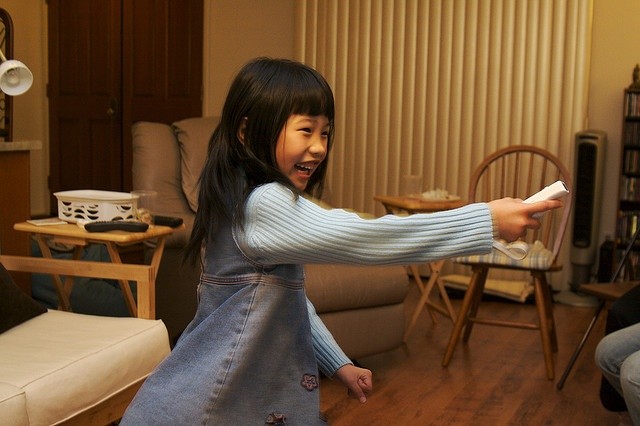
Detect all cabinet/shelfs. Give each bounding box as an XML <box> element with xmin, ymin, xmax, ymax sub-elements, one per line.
<box><xmin>612</xmin><ymin>88</ymin><xmax>640</xmax><ymax>281</ymax></box>
<box><xmin>44</xmin><ymin>1</ymin><xmax>206</xmax><ymax>218</ymax></box>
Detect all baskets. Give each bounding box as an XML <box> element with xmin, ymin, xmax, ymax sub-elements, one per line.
<box><xmin>53</xmin><ymin>189</ymin><xmax>139</xmax><ymax>223</ymax></box>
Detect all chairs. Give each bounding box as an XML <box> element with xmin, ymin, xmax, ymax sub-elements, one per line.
<box><xmin>440</xmin><ymin>145</ymin><xmax>576</xmax><ymax>383</ymax></box>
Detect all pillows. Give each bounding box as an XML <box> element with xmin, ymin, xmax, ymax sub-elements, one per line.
<box><xmin>0</xmin><ymin>258</ymin><xmax>48</xmax><ymax>335</ymax></box>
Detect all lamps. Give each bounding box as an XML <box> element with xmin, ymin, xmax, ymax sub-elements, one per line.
<box><xmin>0</xmin><ymin>49</ymin><xmax>34</xmax><ymax>96</ymax></box>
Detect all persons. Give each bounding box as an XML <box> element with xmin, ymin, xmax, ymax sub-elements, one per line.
<box><xmin>594</xmin><ymin>321</ymin><xmax>640</xmax><ymax>426</ymax></box>
<box><xmin>120</xmin><ymin>56</ymin><xmax>564</xmax><ymax>426</ymax></box>
<box><xmin>599</xmin><ymin>282</ymin><xmax>640</xmax><ymax>413</ymax></box>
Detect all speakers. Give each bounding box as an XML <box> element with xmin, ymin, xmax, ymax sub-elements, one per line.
<box><xmin>568</xmin><ymin>128</ymin><xmax>609</xmax><ymax>293</ymax></box>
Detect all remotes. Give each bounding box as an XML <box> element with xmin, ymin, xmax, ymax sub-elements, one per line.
<box><xmin>84</xmin><ymin>220</ymin><xmax>150</xmax><ymax>234</ymax></box>
<box><xmin>154</xmin><ymin>215</ymin><xmax>183</xmax><ymax>227</ymax></box>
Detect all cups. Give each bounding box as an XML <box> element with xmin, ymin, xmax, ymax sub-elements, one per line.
<box><xmin>130</xmin><ymin>189</ymin><xmax>157</xmax><ymax>230</ymax></box>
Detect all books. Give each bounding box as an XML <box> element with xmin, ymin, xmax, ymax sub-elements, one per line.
<box><xmin>612</xmin><ymin>249</ymin><xmax>640</xmax><ymax>282</ymax></box>
<box><xmin>617</xmin><ymin>208</ymin><xmax>640</xmax><ymax>245</ymax></box>
<box><xmin>624</xmin><ymin>92</ymin><xmax>640</xmax><ymax>117</ymax></box>
<box><xmin>26</xmin><ymin>219</ymin><xmax>68</xmax><ymax>226</ymax></box>
<box><xmin>623</xmin><ymin>149</ymin><xmax>640</xmax><ymax>174</ymax></box>
<box><xmin>620</xmin><ymin>177</ymin><xmax>640</xmax><ymax>201</ymax></box>
<box><xmin>623</xmin><ymin>121</ymin><xmax>640</xmax><ymax>145</ymax></box>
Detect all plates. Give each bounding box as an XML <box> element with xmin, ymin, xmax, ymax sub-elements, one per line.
<box><xmin>406</xmin><ymin>194</ymin><xmax>460</xmax><ymax>203</ymax></box>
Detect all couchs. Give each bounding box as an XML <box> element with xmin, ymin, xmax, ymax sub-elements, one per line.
<box><xmin>0</xmin><ymin>254</ymin><xmax>176</xmax><ymax>426</ymax></box>
<box><xmin>129</xmin><ymin>111</ymin><xmax>411</xmax><ymax>366</ymax></box>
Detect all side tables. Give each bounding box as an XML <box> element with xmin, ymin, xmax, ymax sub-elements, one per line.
<box><xmin>373</xmin><ymin>194</ymin><xmax>468</xmax><ymax>344</ymax></box>
<box><xmin>11</xmin><ymin>212</ymin><xmax>184</xmax><ymax>319</ymax></box>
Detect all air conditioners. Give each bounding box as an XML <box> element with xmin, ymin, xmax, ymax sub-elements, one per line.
<box><xmin>554</xmin><ymin>128</ymin><xmax>608</xmax><ymax>311</ymax></box>
